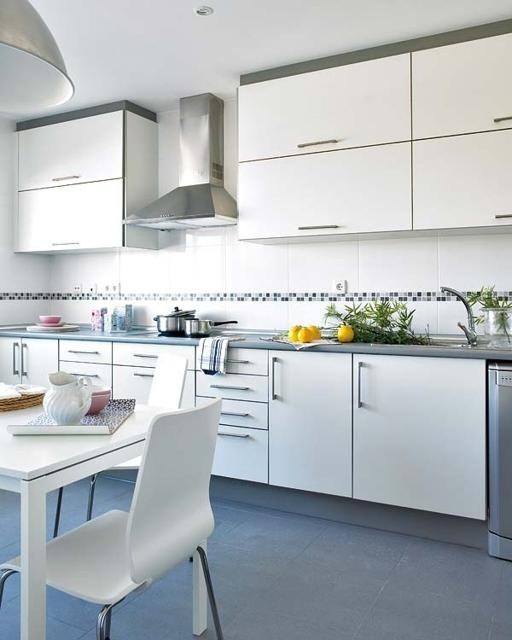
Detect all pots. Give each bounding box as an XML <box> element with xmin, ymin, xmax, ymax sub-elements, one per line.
<box><xmin>153</xmin><ymin>306</ymin><xmax>199</xmax><ymax>337</ymax></box>
<box><xmin>184</xmin><ymin>320</ymin><xmax>238</xmax><ymax>338</ymax></box>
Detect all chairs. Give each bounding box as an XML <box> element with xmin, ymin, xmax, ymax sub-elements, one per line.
<box><xmin>53</xmin><ymin>357</ymin><xmax>188</xmax><ymax>537</ymax></box>
<box><xmin>0</xmin><ymin>398</ymin><xmax>225</xmax><ymax>639</ymax></box>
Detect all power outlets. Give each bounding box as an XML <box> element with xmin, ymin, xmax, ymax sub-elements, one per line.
<box><xmin>333</xmin><ymin>280</ymin><xmax>346</xmax><ymax>294</ymax></box>
<box><xmin>496</xmin><ymin>371</ymin><xmax>512</xmax><ymax>387</ymax></box>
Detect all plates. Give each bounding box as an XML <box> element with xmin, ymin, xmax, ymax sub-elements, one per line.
<box><xmin>38</xmin><ymin>323</ymin><xmax>64</xmax><ymax>327</ymax></box>
<box><xmin>27</xmin><ymin>326</ymin><xmax>80</xmax><ymax>333</ymax></box>
<box><xmin>7</xmin><ymin>398</ymin><xmax>136</xmax><ymax>435</ymax></box>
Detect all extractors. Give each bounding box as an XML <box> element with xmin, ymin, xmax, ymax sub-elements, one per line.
<box><xmin>123</xmin><ymin>93</ymin><xmax>237</xmax><ymax>232</ymax></box>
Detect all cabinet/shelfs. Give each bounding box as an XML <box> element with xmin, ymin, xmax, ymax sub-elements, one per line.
<box><xmin>237</xmin><ymin>18</ymin><xmax>512</xmax><ymax>244</ymax></box>
<box><xmin>15</xmin><ymin>100</ymin><xmax>158</xmax><ymax>255</ymax></box>
<box><xmin>58</xmin><ymin>339</ymin><xmax>112</xmax><ymax>392</ymax></box>
<box><xmin>113</xmin><ymin>342</ymin><xmax>195</xmax><ymax>407</ymax></box>
<box><xmin>195</xmin><ymin>346</ymin><xmax>267</xmax><ymax>485</ymax></box>
<box><xmin>0</xmin><ymin>336</ymin><xmax>59</xmax><ymax>389</ymax></box>
<box><xmin>269</xmin><ymin>350</ymin><xmax>353</xmax><ymax>500</ymax></box>
<box><xmin>352</xmin><ymin>355</ymin><xmax>486</xmax><ymax>521</ymax></box>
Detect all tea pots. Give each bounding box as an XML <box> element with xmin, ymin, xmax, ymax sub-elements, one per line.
<box><xmin>42</xmin><ymin>372</ymin><xmax>93</xmax><ymax>424</ymax></box>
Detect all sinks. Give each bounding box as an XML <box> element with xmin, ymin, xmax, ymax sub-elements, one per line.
<box><xmin>369</xmin><ymin>338</ymin><xmax>490</xmax><ymax>349</ymax></box>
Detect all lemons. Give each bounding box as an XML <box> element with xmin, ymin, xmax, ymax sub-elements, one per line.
<box><xmin>338</xmin><ymin>326</ymin><xmax>354</xmax><ymax>342</ymax></box>
<box><xmin>288</xmin><ymin>325</ymin><xmax>321</xmax><ymax>343</ymax></box>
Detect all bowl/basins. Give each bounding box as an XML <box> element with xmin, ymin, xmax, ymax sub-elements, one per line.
<box><xmin>40</xmin><ymin>316</ymin><xmax>63</xmax><ymax>324</ymax></box>
<box><xmin>86</xmin><ymin>390</ymin><xmax>112</xmax><ymax>416</ymax></box>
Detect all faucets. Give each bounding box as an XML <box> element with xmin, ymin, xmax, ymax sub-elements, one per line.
<box><xmin>442</xmin><ymin>285</ymin><xmax>478</xmax><ymax>346</ymax></box>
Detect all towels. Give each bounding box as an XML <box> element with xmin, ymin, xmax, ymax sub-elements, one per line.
<box><xmin>199</xmin><ymin>336</ymin><xmax>245</xmax><ymax>375</ymax></box>
<box><xmin>259</xmin><ymin>333</ymin><xmax>342</xmax><ymax>351</ymax></box>
